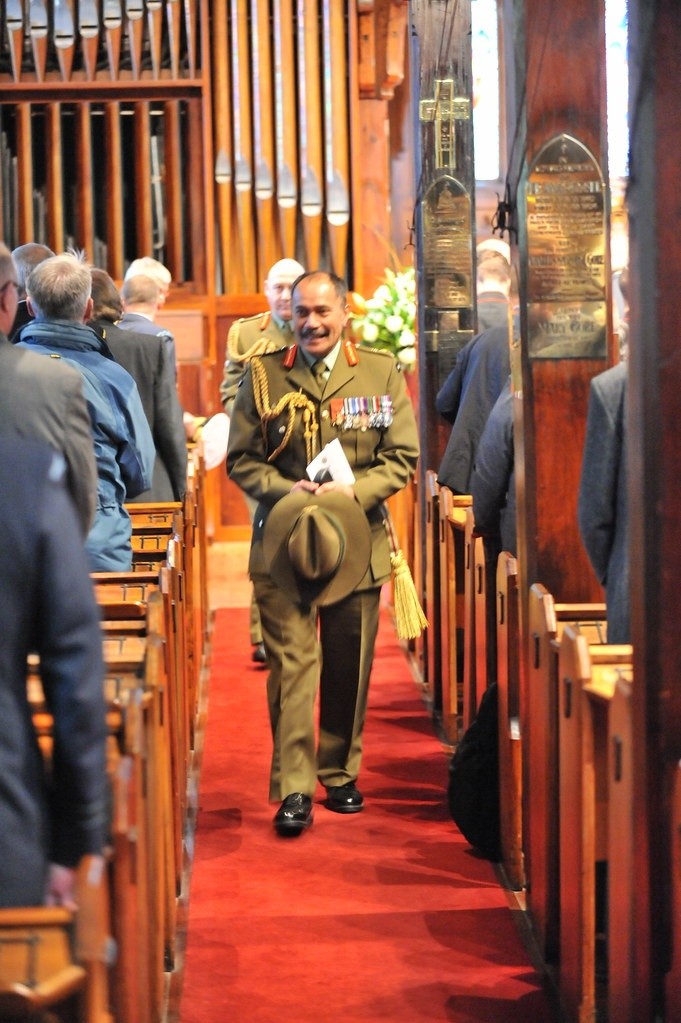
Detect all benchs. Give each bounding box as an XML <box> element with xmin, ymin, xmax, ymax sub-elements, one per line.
<box><xmin>387</xmin><ymin>465</ymin><xmax>681</xmax><ymax>1023</ymax></box>
<box><xmin>0</xmin><ymin>436</ymin><xmax>207</xmax><ymax>1023</ymax></box>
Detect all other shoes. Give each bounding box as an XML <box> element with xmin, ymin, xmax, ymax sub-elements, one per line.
<box><xmin>253</xmin><ymin>642</ymin><xmax>266</xmax><ymax>661</ymax></box>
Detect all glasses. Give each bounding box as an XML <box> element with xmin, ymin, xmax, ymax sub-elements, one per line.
<box><xmin>0</xmin><ymin>281</ymin><xmax>22</xmax><ymax>296</ymax></box>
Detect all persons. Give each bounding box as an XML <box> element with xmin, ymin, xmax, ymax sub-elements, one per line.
<box><xmin>1</xmin><ymin>241</ymin><xmax>187</xmax><ymax>1023</ymax></box>
<box><xmin>219</xmin><ymin>258</ymin><xmax>312</xmax><ymax>662</ymax></box>
<box><xmin>225</xmin><ymin>270</ymin><xmax>421</xmax><ymax>838</ymax></box>
<box><xmin>433</xmin><ymin>232</ymin><xmax>634</xmax><ymax>888</ymax></box>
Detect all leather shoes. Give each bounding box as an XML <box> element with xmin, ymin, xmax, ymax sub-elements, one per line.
<box><xmin>275</xmin><ymin>793</ymin><xmax>313</xmax><ymax>828</ymax></box>
<box><xmin>327</xmin><ymin>780</ymin><xmax>363</xmax><ymax>812</ymax></box>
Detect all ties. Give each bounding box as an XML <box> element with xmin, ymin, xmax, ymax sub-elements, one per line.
<box><xmin>312</xmin><ymin>359</ymin><xmax>327</xmax><ymax>395</ymax></box>
<box><xmin>284</xmin><ymin>321</ymin><xmax>292</xmax><ymax>342</ymax></box>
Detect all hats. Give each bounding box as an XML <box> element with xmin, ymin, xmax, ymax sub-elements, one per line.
<box><xmin>263</xmin><ymin>490</ymin><xmax>373</xmax><ymax>606</ymax></box>
